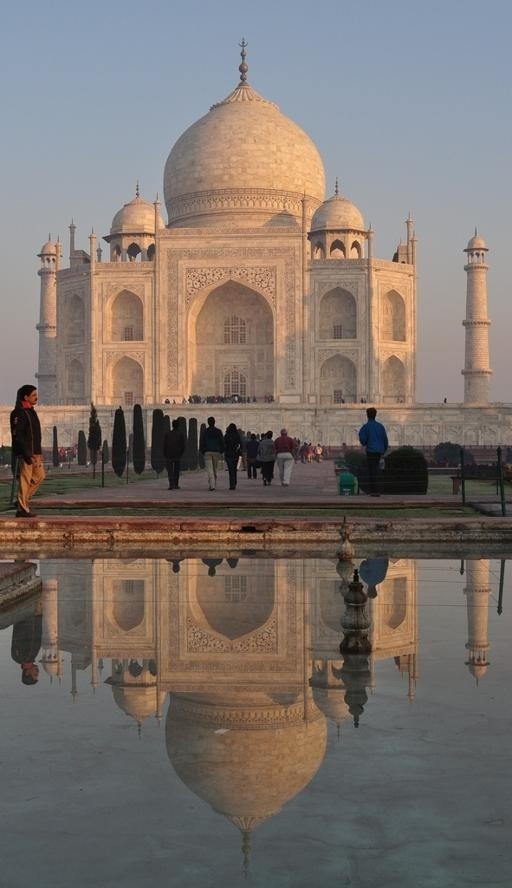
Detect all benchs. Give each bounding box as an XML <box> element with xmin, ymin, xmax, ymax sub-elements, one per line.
<box><xmin>450</xmin><ymin>463</ymin><xmax>504</xmax><ymax>496</ymax></box>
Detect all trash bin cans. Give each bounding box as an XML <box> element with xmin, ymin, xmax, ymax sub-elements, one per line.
<box><xmin>339</xmin><ymin>472</ymin><xmax>354</xmax><ymax>496</ymax></box>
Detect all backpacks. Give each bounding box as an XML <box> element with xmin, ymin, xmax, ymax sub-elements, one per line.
<box><xmin>229</xmin><ymin>435</ymin><xmax>242</xmax><ymax>458</ymax></box>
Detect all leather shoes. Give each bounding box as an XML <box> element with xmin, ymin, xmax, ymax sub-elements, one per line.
<box><xmin>16</xmin><ymin>510</ymin><xmax>37</xmax><ymax>517</ymax></box>
<box><xmin>13</xmin><ymin>500</ymin><xmax>32</xmax><ymax>510</ymax></box>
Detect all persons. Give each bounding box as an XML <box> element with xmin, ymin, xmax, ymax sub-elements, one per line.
<box><xmin>165</xmin><ymin>393</ymin><xmax>274</xmax><ymax>404</ymax></box>
<box><xmin>10</xmin><ymin>384</ymin><xmax>46</xmax><ymax>518</ymax></box>
<box><xmin>342</xmin><ymin>442</ymin><xmax>347</xmax><ymax>451</ymax></box>
<box><xmin>226</xmin><ymin>423</ymin><xmax>329</xmax><ymax>489</ymax></box>
<box><xmin>359</xmin><ymin>557</ymin><xmax>389</xmax><ymax>598</ymax></box>
<box><xmin>60</xmin><ymin>446</ymin><xmax>65</xmax><ymax>462</ymax></box>
<box><xmin>200</xmin><ymin>416</ymin><xmax>225</xmax><ymax>492</ymax></box>
<box><xmin>10</xmin><ymin>613</ymin><xmax>44</xmax><ymax>687</ymax></box>
<box><xmin>162</xmin><ymin>419</ymin><xmax>186</xmax><ymax>491</ymax></box>
<box><xmin>358</xmin><ymin>406</ymin><xmax>389</xmax><ymax>498</ymax></box>
